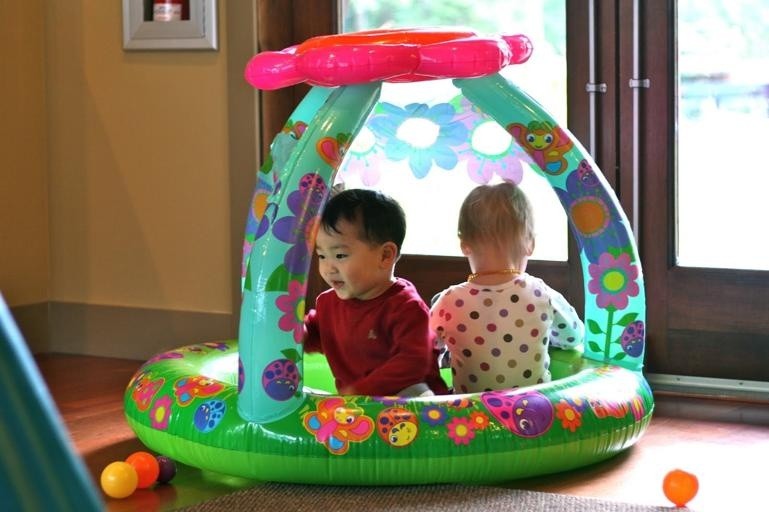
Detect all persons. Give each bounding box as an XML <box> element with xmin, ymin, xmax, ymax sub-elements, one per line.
<box><xmin>303</xmin><ymin>188</ymin><xmax>451</xmax><ymax>401</ymax></box>
<box><xmin>428</xmin><ymin>178</ymin><xmax>589</xmax><ymax>396</ymax></box>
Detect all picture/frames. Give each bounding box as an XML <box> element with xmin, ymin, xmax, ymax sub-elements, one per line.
<box><xmin>121</xmin><ymin>1</ymin><xmax>220</xmax><ymax>53</ymax></box>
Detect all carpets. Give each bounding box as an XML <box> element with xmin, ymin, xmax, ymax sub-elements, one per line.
<box><xmin>176</xmin><ymin>481</ymin><xmax>691</xmax><ymax>511</ymax></box>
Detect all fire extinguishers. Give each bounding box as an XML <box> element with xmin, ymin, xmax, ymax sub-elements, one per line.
<box><xmin>152</xmin><ymin>0</ymin><xmax>188</xmax><ymax>22</ymax></box>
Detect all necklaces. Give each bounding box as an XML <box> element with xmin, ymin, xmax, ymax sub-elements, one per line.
<box><xmin>466</xmin><ymin>267</ymin><xmax>524</xmax><ymax>285</ymax></box>
